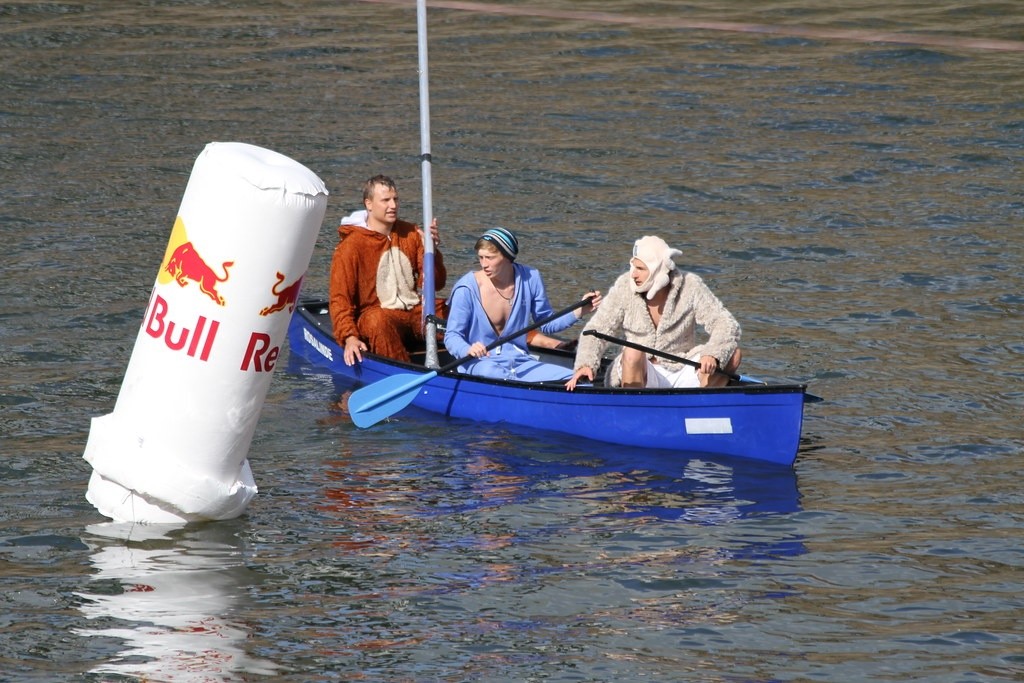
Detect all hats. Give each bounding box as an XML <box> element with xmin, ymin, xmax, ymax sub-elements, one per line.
<box><xmin>628</xmin><ymin>235</ymin><xmax>683</xmax><ymax>299</ymax></box>
<box><xmin>480</xmin><ymin>226</ymin><xmax>518</xmax><ymax>262</ymax></box>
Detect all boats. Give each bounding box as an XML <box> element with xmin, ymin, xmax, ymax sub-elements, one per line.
<box><xmin>289</xmin><ymin>296</ymin><xmax>807</xmax><ymax>467</ymax></box>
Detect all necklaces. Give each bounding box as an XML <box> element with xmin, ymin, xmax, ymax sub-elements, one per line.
<box><xmin>490</xmin><ymin>279</ymin><xmax>514</xmax><ymax>306</ymax></box>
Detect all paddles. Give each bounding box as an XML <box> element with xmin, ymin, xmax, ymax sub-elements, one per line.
<box><xmin>580</xmin><ymin>330</ymin><xmax>825</xmax><ymax>405</ymax></box>
<box><xmin>346</xmin><ymin>288</ymin><xmax>598</xmax><ymax>429</ymax></box>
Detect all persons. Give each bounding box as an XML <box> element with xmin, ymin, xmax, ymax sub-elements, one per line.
<box><xmin>329</xmin><ymin>175</ymin><xmax>451</xmax><ymax>366</ymax></box>
<box><xmin>565</xmin><ymin>236</ymin><xmax>742</xmax><ymax>391</ymax></box>
<box><xmin>443</xmin><ymin>227</ymin><xmax>603</xmax><ymax>381</ymax></box>
